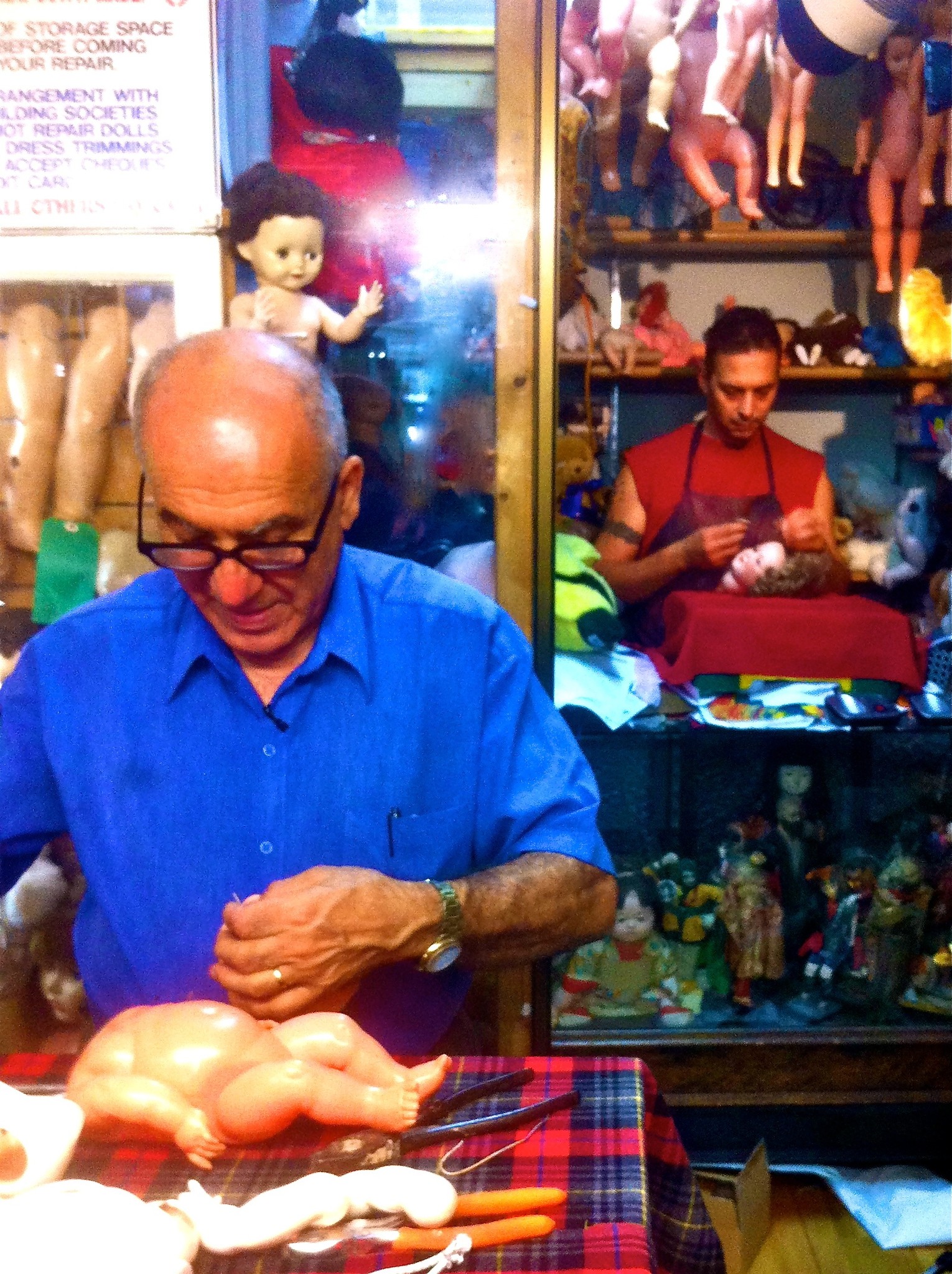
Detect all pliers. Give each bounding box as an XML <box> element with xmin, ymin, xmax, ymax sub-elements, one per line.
<box><xmin>307</xmin><ymin>1068</ymin><xmax>581</xmax><ymax>1174</ymax></box>
<box><xmin>281</xmin><ymin>1187</ymin><xmax>572</xmax><ymax>1259</ymax></box>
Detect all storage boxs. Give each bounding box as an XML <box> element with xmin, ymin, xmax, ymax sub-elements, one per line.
<box><xmin>696</xmin><ymin>1134</ymin><xmax>773</xmax><ymax>1273</ymax></box>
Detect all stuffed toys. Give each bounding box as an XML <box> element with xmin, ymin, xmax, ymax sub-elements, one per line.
<box><xmin>554</xmin><ymin>435</ymin><xmax>607</xmax><ymax>522</ymax></box>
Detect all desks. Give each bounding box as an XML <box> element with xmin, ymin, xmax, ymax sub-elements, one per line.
<box><xmin>0</xmin><ymin>1050</ymin><xmax>730</xmax><ymax>1274</ymax></box>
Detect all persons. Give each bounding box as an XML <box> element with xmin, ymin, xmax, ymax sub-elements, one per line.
<box><xmin>776</xmin><ymin>310</ymin><xmax>900</xmax><ymax>366</ymax></box>
<box><xmin>594</xmin><ymin>309</ymin><xmax>849</xmax><ymax>648</ymax></box>
<box><xmin>722</xmin><ymin>542</ymin><xmax>829</xmax><ymax>598</ymax></box>
<box><xmin>556</xmin><ymin>0</ymin><xmax>951</xmax><ymax>292</ymax></box>
<box><xmin>229</xmin><ymin>163</ymin><xmax>384</xmax><ymax>364</ymax></box>
<box><xmin>602</xmin><ymin>280</ymin><xmax>686</xmax><ymax>374</ymax></box>
<box><xmin>549</xmin><ymin>768</ymin><xmax>952</xmax><ymax>1028</ymax></box>
<box><xmin>1</xmin><ymin>281</ymin><xmax>129</xmax><ymax>549</ymax></box>
<box><xmin>64</xmin><ymin>1004</ymin><xmax>450</xmax><ymax>1169</ymax></box>
<box><xmin>0</xmin><ymin>328</ymin><xmax>619</xmax><ymax>1055</ymax></box>
<box><xmin>281</xmin><ymin>35</ymin><xmax>419</xmax><ymax>446</ymax></box>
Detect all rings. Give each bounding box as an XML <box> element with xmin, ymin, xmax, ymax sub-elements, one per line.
<box><xmin>272</xmin><ymin>966</ymin><xmax>288</xmax><ymax>991</ymax></box>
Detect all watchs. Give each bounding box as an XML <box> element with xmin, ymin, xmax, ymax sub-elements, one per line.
<box><xmin>413</xmin><ymin>880</ymin><xmax>464</xmax><ymax>972</ymax></box>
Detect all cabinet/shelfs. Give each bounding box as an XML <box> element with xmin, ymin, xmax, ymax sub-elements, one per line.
<box><xmin>466</xmin><ymin>226</ymin><xmax>952</xmax><ymax>381</ymax></box>
<box><xmin>549</xmin><ymin>709</ymin><xmax>951</xmax><ymax>1111</ymax></box>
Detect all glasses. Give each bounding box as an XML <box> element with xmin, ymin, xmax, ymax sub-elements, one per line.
<box><xmin>136</xmin><ymin>466</ymin><xmax>339</xmax><ymax>572</ymax></box>
